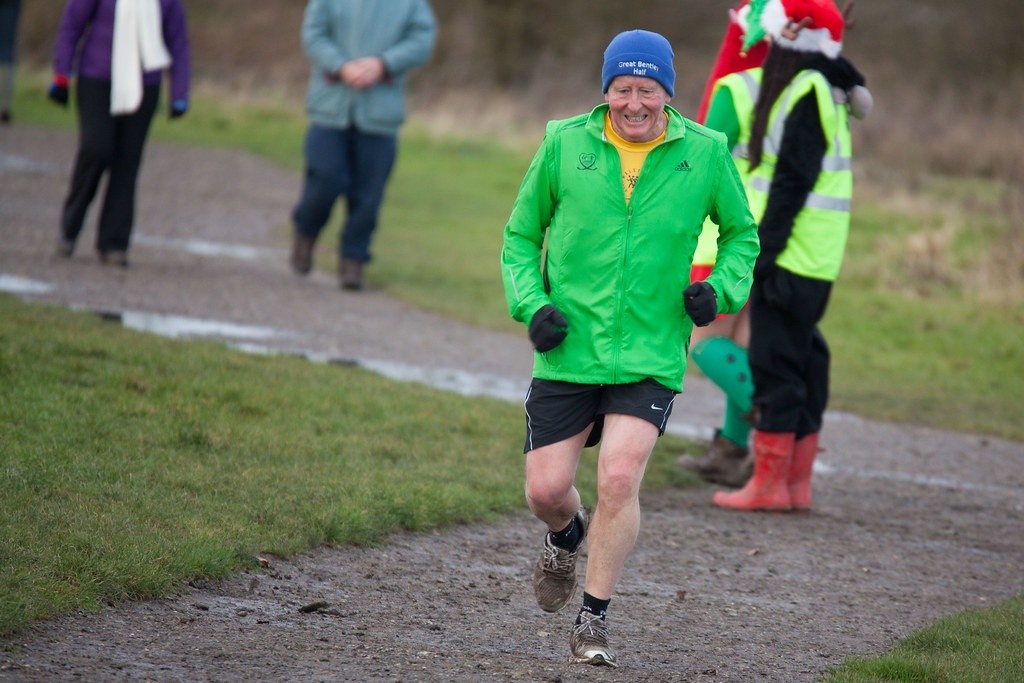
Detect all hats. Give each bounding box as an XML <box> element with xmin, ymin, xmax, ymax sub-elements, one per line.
<box><xmin>600</xmin><ymin>29</ymin><xmax>676</xmax><ymax>97</ymax></box>
<box><xmin>760</xmin><ymin>0</ymin><xmax>844</xmax><ymax>61</ymax></box>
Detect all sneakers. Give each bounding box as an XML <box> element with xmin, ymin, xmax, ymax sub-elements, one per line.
<box><xmin>532</xmin><ymin>506</ymin><xmax>589</xmax><ymax>613</ymax></box>
<box><xmin>568</xmin><ymin>611</ymin><xmax>619</xmax><ymax>668</ymax></box>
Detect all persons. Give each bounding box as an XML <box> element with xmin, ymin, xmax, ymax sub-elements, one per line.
<box><xmin>295</xmin><ymin>1</ymin><xmax>440</xmax><ymax>284</ymax></box>
<box><xmin>680</xmin><ymin>0</ymin><xmax>771</xmax><ymax>481</ymax></box>
<box><xmin>48</xmin><ymin>1</ymin><xmax>189</xmax><ymax>266</ymax></box>
<box><xmin>501</xmin><ymin>29</ymin><xmax>760</xmax><ymax>657</ymax></box>
<box><xmin>713</xmin><ymin>0</ymin><xmax>872</xmax><ymax>511</ymax></box>
<box><xmin>0</xmin><ymin>0</ymin><xmax>23</xmax><ymax>121</ymax></box>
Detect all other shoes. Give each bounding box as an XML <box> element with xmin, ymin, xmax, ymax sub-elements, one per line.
<box><xmin>101</xmin><ymin>248</ymin><xmax>128</xmax><ymax>265</ymax></box>
<box><xmin>336</xmin><ymin>258</ymin><xmax>362</xmax><ymax>288</ymax></box>
<box><xmin>677</xmin><ymin>429</ymin><xmax>752</xmax><ymax>488</ymax></box>
<box><xmin>291</xmin><ymin>234</ymin><xmax>315</xmax><ymax>275</ymax></box>
<box><xmin>55</xmin><ymin>237</ymin><xmax>77</xmax><ymax>257</ymax></box>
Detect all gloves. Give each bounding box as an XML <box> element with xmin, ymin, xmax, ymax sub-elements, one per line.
<box><xmin>48</xmin><ymin>85</ymin><xmax>68</xmax><ymax>105</ymax></box>
<box><xmin>684</xmin><ymin>281</ymin><xmax>716</xmax><ymax>328</ymax></box>
<box><xmin>527</xmin><ymin>304</ymin><xmax>568</xmax><ymax>353</ymax></box>
<box><xmin>169</xmin><ymin>98</ymin><xmax>186</xmax><ymax>119</ymax></box>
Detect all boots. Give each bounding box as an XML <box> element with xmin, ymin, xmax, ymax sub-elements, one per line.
<box><xmin>712</xmin><ymin>432</ymin><xmax>794</xmax><ymax>511</ymax></box>
<box><xmin>788</xmin><ymin>433</ymin><xmax>818</xmax><ymax>509</ymax></box>
